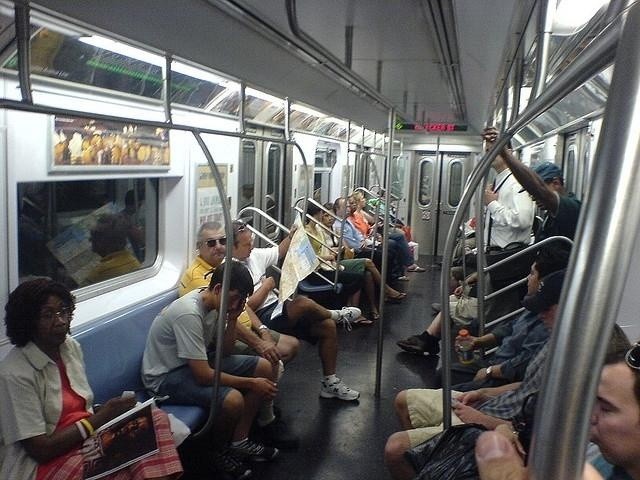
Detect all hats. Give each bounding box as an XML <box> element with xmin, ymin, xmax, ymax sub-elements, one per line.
<box><xmin>521</xmin><ymin>267</ymin><xmax>565</xmax><ymax>310</ymax></box>
<box><xmin>518</xmin><ymin>162</ymin><xmax>563</xmax><ymax>194</ymax></box>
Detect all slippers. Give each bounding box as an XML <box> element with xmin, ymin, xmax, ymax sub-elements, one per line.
<box><xmin>408</xmin><ymin>267</ymin><xmax>425</xmax><ymax>272</ymax></box>
<box><xmin>371</xmin><ymin>312</ymin><xmax>380</xmax><ymax>319</ymax></box>
<box><xmin>399</xmin><ymin>276</ymin><xmax>410</xmax><ymax>280</ymax></box>
<box><xmin>388</xmin><ymin>293</ymin><xmax>407</xmax><ymax>300</ymax></box>
<box><xmin>354</xmin><ymin>319</ymin><xmax>374</xmax><ymax>326</ymax></box>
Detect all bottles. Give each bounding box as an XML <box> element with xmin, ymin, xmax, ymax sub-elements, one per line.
<box><xmin>456</xmin><ymin>330</ymin><xmax>475</xmax><ymax>364</ymax></box>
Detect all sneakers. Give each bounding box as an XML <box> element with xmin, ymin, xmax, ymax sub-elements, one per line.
<box><xmin>432</xmin><ymin>303</ymin><xmax>442</xmax><ymax>311</ymax></box>
<box><xmin>320</xmin><ymin>379</ymin><xmax>360</xmax><ymax>400</ymax></box>
<box><xmin>229</xmin><ymin>442</ymin><xmax>279</xmax><ymax>461</ymax></box>
<box><xmin>208</xmin><ymin>450</ymin><xmax>251</xmax><ymax>478</ymax></box>
<box><xmin>336</xmin><ymin>307</ymin><xmax>361</xmax><ymax>331</ymax></box>
<box><xmin>396</xmin><ymin>336</ymin><xmax>426</xmax><ymax>352</ymax></box>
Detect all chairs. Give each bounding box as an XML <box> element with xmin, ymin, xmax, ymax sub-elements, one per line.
<box><xmin>297</xmin><ymin>274</ymin><xmax>341</xmax><ymax>307</ymax></box>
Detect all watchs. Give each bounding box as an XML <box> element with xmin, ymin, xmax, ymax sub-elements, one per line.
<box><xmin>256</xmin><ymin>323</ymin><xmax>268</xmax><ymax>333</ymax></box>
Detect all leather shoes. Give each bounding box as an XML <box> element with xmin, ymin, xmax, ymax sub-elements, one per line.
<box><xmin>257</xmin><ymin>405</ymin><xmax>281</xmax><ymax>418</ymax></box>
<box><xmin>254</xmin><ymin>418</ymin><xmax>300</xmax><ymax>447</ymax></box>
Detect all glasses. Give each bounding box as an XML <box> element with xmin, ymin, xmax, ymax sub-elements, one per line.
<box><xmin>625</xmin><ymin>341</ymin><xmax>640</xmax><ymax>368</ymax></box>
<box><xmin>530</xmin><ymin>194</ymin><xmax>536</xmax><ymax>199</ymax></box>
<box><xmin>205</xmin><ymin>238</ymin><xmax>226</xmax><ymax>247</ymax></box>
<box><xmin>234</xmin><ymin>223</ymin><xmax>246</xmax><ymax>233</ymax></box>
<box><xmin>39</xmin><ymin>306</ymin><xmax>70</xmax><ymax>321</ymax></box>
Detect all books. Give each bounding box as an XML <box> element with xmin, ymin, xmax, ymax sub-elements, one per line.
<box><xmin>83</xmin><ymin>395</ymin><xmax>171</xmax><ymax>480</ymax></box>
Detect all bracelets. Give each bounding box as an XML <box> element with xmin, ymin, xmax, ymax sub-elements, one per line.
<box><xmin>75</xmin><ymin>419</ymin><xmax>93</xmax><ymax>439</ymax></box>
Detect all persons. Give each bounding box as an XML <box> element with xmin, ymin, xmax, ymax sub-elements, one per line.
<box><xmin>141</xmin><ymin>261</ymin><xmax>280</xmax><ymax>478</ymax></box>
<box><xmin>431</xmin><ymin>227</ymin><xmax>479</xmax><ymax>311</ymax></box>
<box><xmin>376</xmin><ymin>190</ymin><xmax>413</xmax><ymax>244</ymax></box>
<box><xmin>316</xmin><ymin>202</ymin><xmax>354</xmax><ymax>261</ymax></box>
<box><xmin>386</xmin><ymin>266</ymin><xmax>567</xmax><ymax>480</ymax></box>
<box><xmin>178</xmin><ymin>223</ymin><xmax>302</xmax><ymax>447</ymax></box>
<box><xmin>396</xmin><ymin>243</ymin><xmax>536</xmax><ymax>355</ymax></box>
<box><xmin>586</xmin><ymin>343</ymin><xmax>640</xmax><ymax>480</ymax></box>
<box><xmin>303</xmin><ymin>202</ymin><xmax>409</xmax><ymax>319</ymax></box>
<box><xmin>121</xmin><ymin>189</ymin><xmax>145</xmax><ymax>260</ymax></box>
<box><xmin>397</xmin><ymin>286</ymin><xmax>478</xmax><ymax>354</ymax></box>
<box><xmin>483</xmin><ymin>126</ymin><xmax>583</xmax><ymax>271</ymax></box>
<box><xmin>80</xmin><ymin>213</ymin><xmax>144</xmax><ymax>288</ymax></box>
<box><xmin>302</xmin><ymin>204</ymin><xmax>374</xmax><ymax>325</ymax></box>
<box><xmin>352</xmin><ymin>190</ymin><xmax>427</xmax><ymax>281</ymax></box>
<box><xmin>347</xmin><ymin>195</ymin><xmax>382</xmax><ymax>240</ymax></box>
<box><xmin>484</xmin><ymin>135</ymin><xmax>535</xmax><ymax>305</ymax></box>
<box><xmin>232</xmin><ymin>218</ymin><xmax>361</xmax><ymax>401</ymax></box>
